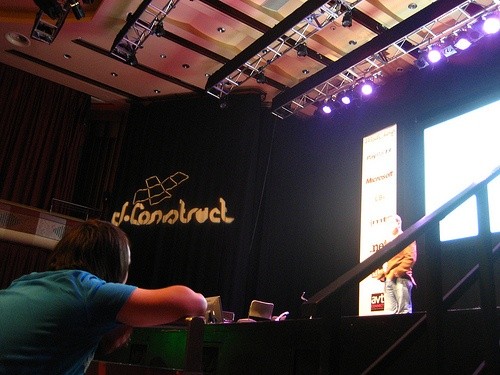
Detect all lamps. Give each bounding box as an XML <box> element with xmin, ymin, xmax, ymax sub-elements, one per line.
<box><xmin>155</xmin><ymin>24</ymin><xmax>165</xmax><ymax>36</ymax></box>
<box><xmin>297</xmin><ymin>44</ymin><xmax>308</xmax><ymax>57</ymax></box>
<box><xmin>342</xmin><ymin>12</ymin><xmax>352</xmax><ymax>27</ymax></box>
<box><xmin>412</xmin><ymin>14</ymin><xmax>500</xmax><ymax>72</ymax></box>
<box><xmin>220</xmin><ymin>97</ymin><xmax>227</xmax><ymax>110</ymax></box>
<box><xmin>127</xmin><ymin>53</ymin><xmax>137</xmax><ymax>66</ymax></box>
<box><xmin>320</xmin><ymin>79</ymin><xmax>374</xmax><ymax>114</ymax></box>
<box><xmin>69</xmin><ymin>0</ymin><xmax>85</xmax><ymax>21</ymax></box>
<box><xmin>257</xmin><ymin>72</ymin><xmax>266</xmax><ymax>83</ymax></box>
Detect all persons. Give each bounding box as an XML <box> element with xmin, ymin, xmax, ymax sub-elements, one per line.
<box><xmin>377</xmin><ymin>215</ymin><xmax>417</xmax><ymax>315</ymax></box>
<box><xmin>0</xmin><ymin>219</ymin><xmax>208</xmax><ymax>375</ymax></box>
